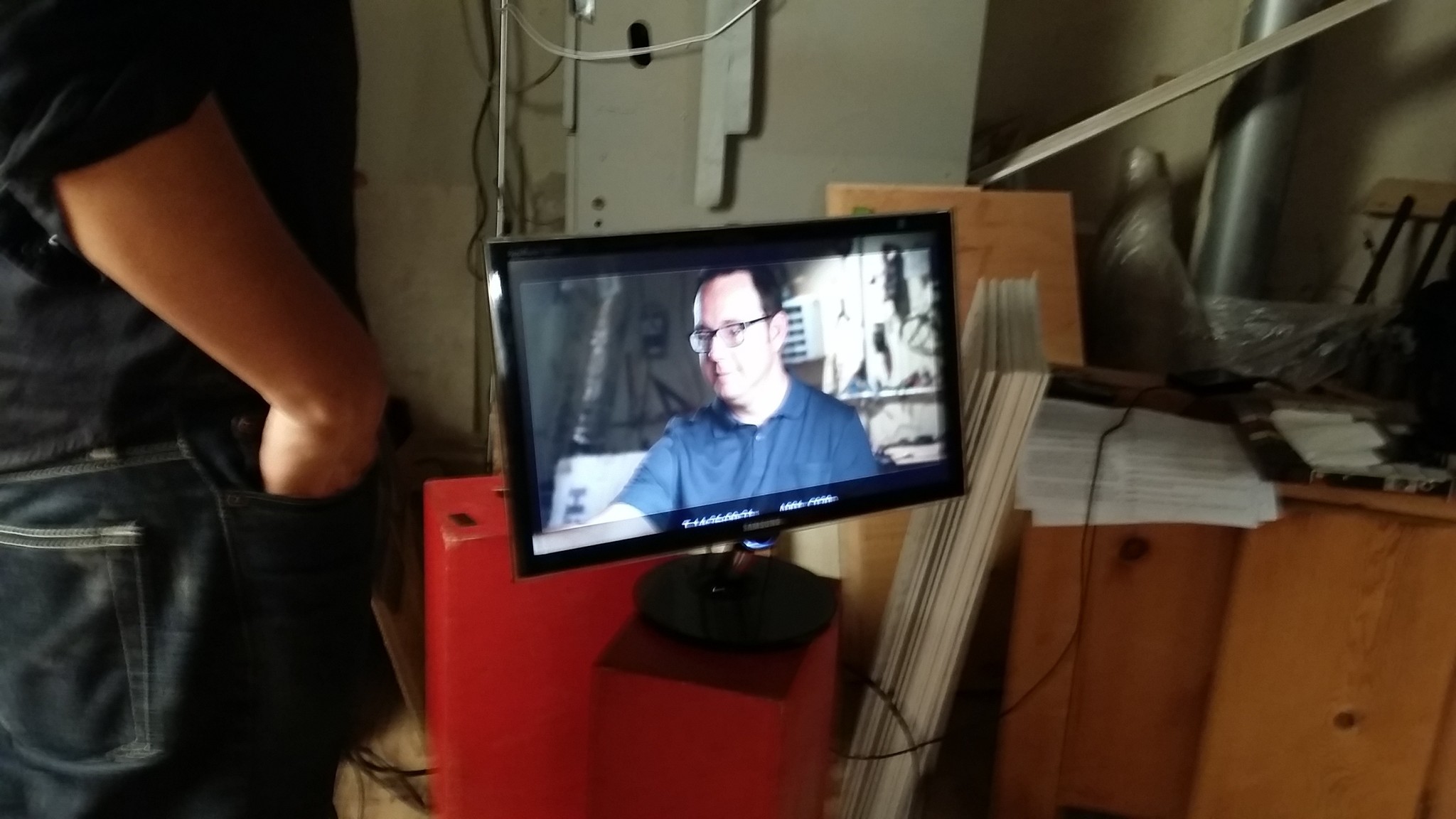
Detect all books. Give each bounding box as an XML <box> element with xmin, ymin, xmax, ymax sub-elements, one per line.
<box><xmin>832</xmin><ymin>278</ymin><xmax>1050</xmax><ymax>819</ymax></box>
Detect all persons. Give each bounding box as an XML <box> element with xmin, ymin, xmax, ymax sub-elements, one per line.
<box><xmin>0</xmin><ymin>0</ymin><xmax>398</xmax><ymax>819</ymax></box>
<box><xmin>529</xmin><ymin>264</ymin><xmax>880</xmax><ymax>533</ymax></box>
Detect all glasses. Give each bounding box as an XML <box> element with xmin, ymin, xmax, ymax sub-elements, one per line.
<box><xmin>686</xmin><ymin>314</ymin><xmax>772</xmax><ymax>354</ymax></box>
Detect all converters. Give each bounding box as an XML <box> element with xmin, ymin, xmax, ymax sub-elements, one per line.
<box><xmin>1165</xmin><ymin>369</ymin><xmax>1252</xmax><ymax>394</ymax></box>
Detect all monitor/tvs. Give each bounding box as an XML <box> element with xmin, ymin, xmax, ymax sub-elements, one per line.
<box><xmin>482</xmin><ymin>209</ymin><xmax>967</xmax><ymax>650</ymax></box>
